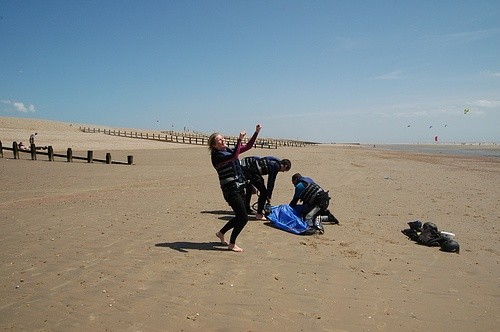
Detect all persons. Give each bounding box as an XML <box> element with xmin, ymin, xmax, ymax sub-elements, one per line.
<box><xmin>18</xmin><ymin>142</ymin><xmax>26</xmax><ymax>149</ymax></box>
<box><xmin>288</xmin><ymin>172</ymin><xmax>339</xmax><ymax>224</ymax></box>
<box><xmin>30</xmin><ymin>132</ymin><xmax>38</xmax><ymax>145</ymax></box>
<box><xmin>240</xmin><ymin>157</ymin><xmax>261</xmax><ymax>215</ymax></box>
<box><xmin>208</xmin><ymin>123</ymin><xmax>262</xmax><ymax>251</ymax></box>
<box><xmin>248</xmin><ymin>156</ymin><xmax>291</xmax><ymax>221</ymax></box>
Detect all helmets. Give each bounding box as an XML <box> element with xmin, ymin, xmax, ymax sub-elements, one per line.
<box><xmin>439</xmin><ymin>240</ymin><xmax>459</xmax><ymax>252</ymax></box>
<box><xmin>423</xmin><ymin>222</ymin><xmax>437</xmax><ymax>231</ymax></box>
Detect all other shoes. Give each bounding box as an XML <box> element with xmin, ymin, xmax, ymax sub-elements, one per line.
<box><xmin>313</xmin><ymin>215</ymin><xmax>324</xmax><ymax>233</ymax></box>
<box><xmin>328</xmin><ymin>214</ymin><xmax>339</xmax><ymax>223</ymax></box>
